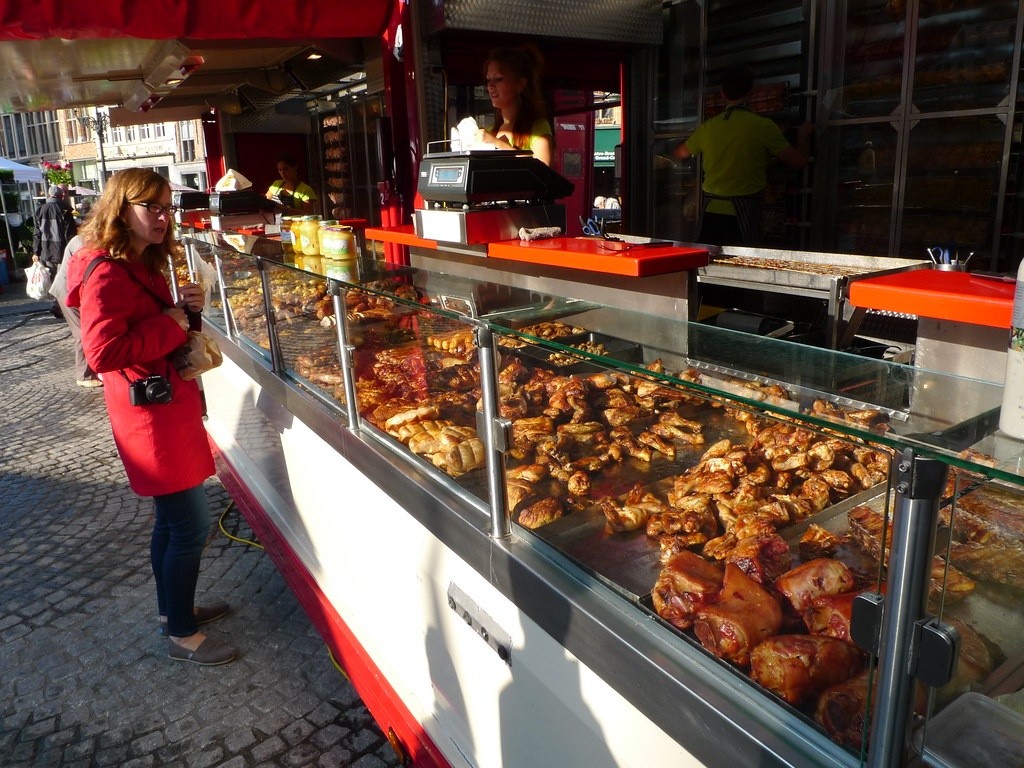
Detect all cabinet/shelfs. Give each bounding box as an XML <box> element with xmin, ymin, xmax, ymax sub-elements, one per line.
<box><xmin>642</xmin><ymin>0</ymin><xmax>1024</xmax><ymax>274</ymax></box>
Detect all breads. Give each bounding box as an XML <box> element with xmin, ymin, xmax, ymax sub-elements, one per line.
<box><xmin>385</xmin><ymin>405</ymin><xmax>486</xmax><ymax>477</ymax></box>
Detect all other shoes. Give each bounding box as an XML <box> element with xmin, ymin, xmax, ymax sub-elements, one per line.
<box><xmin>159</xmin><ymin>600</ymin><xmax>231</xmax><ymax>636</ymax></box>
<box><xmin>165</xmin><ymin>634</ymin><xmax>238</xmax><ymax>666</ymax></box>
<box><xmin>50</xmin><ymin>306</ymin><xmax>63</xmax><ymax>318</ymax></box>
<box><xmin>77</xmin><ymin>379</ymin><xmax>103</xmax><ymax>388</ymax></box>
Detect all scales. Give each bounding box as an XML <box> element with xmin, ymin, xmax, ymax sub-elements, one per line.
<box><xmin>209</xmin><ymin>187</ymin><xmax>281</xmax><ymax>217</ymax></box>
<box><xmin>174</xmin><ymin>188</ymin><xmax>209</xmax><ymax>212</ymax></box>
<box><xmin>417</xmin><ymin>139</ymin><xmax>575</xmax><ymax>211</ymax></box>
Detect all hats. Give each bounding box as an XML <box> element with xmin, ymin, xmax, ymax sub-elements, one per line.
<box><xmin>48</xmin><ymin>185</ymin><xmax>63</xmax><ymax>195</ymax></box>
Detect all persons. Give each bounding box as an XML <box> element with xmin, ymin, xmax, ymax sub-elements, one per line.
<box><xmin>64</xmin><ymin>168</ymin><xmax>238</xmax><ymax>665</ymax></box>
<box><xmin>57</xmin><ymin>182</ymin><xmax>70</xmax><ymax>206</ymax></box>
<box><xmin>31</xmin><ymin>185</ymin><xmax>81</xmax><ymax>319</ymax></box>
<box><xmin>475</xmin><ymin>45</ymin><xmax>556</xmax><ymax>166</ymax></box>
<box><xmin>259</xmin><ymin>153</ymin><xmax>319</xmax><ymax>226</ymax></box>
<box><xmin>48</xmin><ymin>234</ymin><xmax>105</xmax><ymax>388</ymax></box>
<box><xmin>72</xmin><ymin>198</ymin><xmax>92</xmax><ymax>227</ymax></box>
<box><xmin>593</xmin><ymin>195</ymin><xmax>621</xmax><ymax>210</ymax></box>
<box><xmin>671</xmin><ymin>63</ymin><xmax>817</xmax><ymax>250</ymax></box>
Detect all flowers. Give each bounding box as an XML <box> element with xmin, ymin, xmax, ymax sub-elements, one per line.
<box><xmin>40</xmin><ymin>160</ymin><xmax>75</xmax><ymax>184</ymax></box>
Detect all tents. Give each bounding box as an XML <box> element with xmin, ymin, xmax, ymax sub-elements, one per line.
<box><xmin>0</xmin><ymin>157</ymin><xmax>51</xmax><ymax>280</ymax></box>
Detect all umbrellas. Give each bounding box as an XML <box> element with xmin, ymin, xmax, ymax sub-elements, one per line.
<box><xmin>70</xmin><ymin>184</ymin><xmax>103</xmax><ymax>198</ymax></box>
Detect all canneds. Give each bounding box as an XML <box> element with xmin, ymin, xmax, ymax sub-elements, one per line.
<box><xmin>280</xmin><ymin>214</ymin><xmax>357</xmax><ymax>261</ymax></box>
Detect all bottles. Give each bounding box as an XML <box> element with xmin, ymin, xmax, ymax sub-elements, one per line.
<box><xmin>322</xmin><ymin>224</ymin><xmax>344</xmax><ymax>258</ymax></box>
<box><xmin>280</xmin><ymin>215</ymin><xmax>297</xmax><ymax>254</ymax></box>
<box><xmin>291</xmin><ymin>217</ymin><xmax>304</xmax><ymax>255</ymax></box>
<box><xmin>332</xmin><ymin>226</ymin><xmax>357</xmax><ymax>260</ymax></box>
<box><xmin>317</xmin><ymin>219</ymin><xmax>339</xmax><ymax>256</ymax></box>
<box><xmin>300</xmin><ymin>214</ymin><xmax>322</xmax><ymax>256</ymax></box>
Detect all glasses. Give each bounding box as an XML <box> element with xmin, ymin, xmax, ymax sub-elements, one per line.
<box><xmin>123</xmin><ymin>200</ymin><xmax>177</xmax><ymax>216</ymax></box>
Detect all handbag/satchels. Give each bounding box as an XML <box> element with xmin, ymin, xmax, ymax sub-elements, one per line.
<box><xmin>215</xmin><ymin>169</ymin><xmax>252</xmax><ymax>191</ymax></box>
<box><xmin>175</xmin><ymin>330</ymin><xmax>223</xmax><ymax>382</ymax></box>
<box><xmin>23</xmin><ymin>261</ymin><xmax>52</xmax><ymax>302</ymax></box>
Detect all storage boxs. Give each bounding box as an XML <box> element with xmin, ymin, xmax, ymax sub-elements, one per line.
<box><xmin>155</xmin><ymin>224</ymin><xmax>1023</xmax><ymax>766</ymax></box>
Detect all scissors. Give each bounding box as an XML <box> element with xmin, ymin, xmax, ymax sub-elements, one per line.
<box><xmin>931</xmin><ymin>247</ymin><xmax>949</xmax><ymax>264</ymax></box>
<box><xmin>582</xmin><ymin>218</ymin><xmax>600</xmax><ymax>235</ymax></box>
